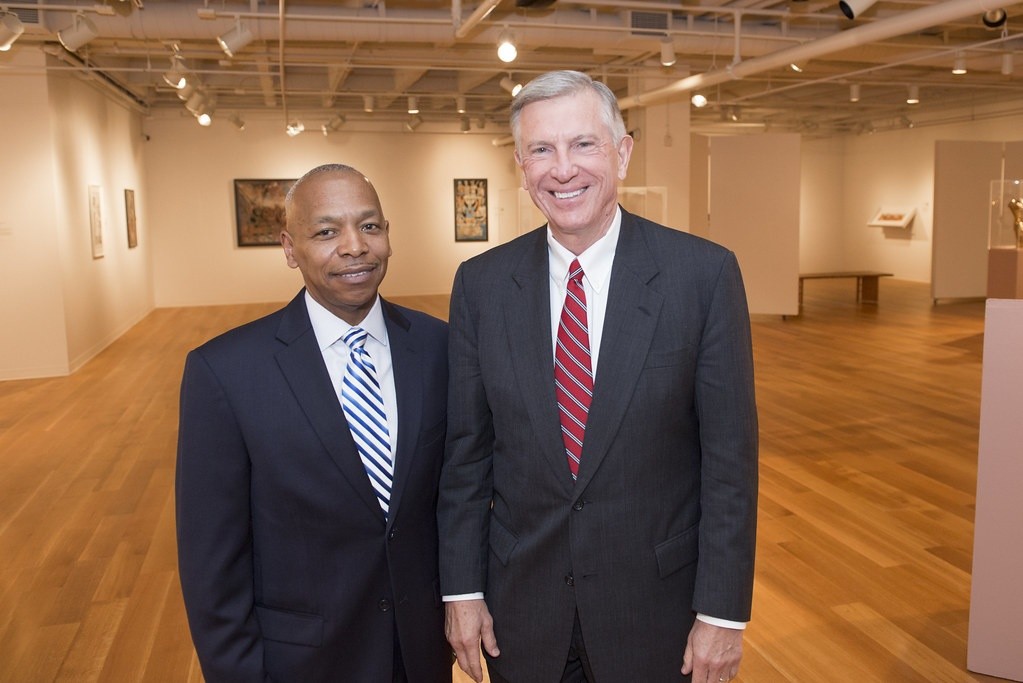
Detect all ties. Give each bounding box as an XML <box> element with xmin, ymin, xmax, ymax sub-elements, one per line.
<box><xmin>341</xmin><ymin>328</ymin><xmax>392</xmax><ymax>521</ymax></box>
<box><xmin>554</xmin><ymin>260</ymin><xmax>594</xmax><ymax>484</ymax></box>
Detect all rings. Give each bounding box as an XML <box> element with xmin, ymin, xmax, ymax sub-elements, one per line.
<box><xmin>720</xmin><ymin>677</ymin><xmax>730</xmax><ymax>682</ymax></box>
<box><xmin>452</xmin><ymin>652</ymin><xmax>458</xmax><ymax>660</ymax></box>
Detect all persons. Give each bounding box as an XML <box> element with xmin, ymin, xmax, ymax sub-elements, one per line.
<box><xmin>177</xmin><ymin>164</ymin><xmax>452</xmax><ymax>683</ymax></box>
<box><xmin>435</xmin><ymin>69</ymin><xmax>757</xmax><ymax>683</ymax></box>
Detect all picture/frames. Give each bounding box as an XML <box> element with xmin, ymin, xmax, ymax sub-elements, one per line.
<box><xmin>866</xmin><ymin>206</ymin><xmax>916</xmax><ymax>229</ymax></box>
<box><xmin>125</xmin><ymin>189</ymin><xmax>138</xmax><ymax>248</ymax></box>
<box><xmin>453</xmin><ymin>178</ymin><xmax>488</xmax><ymax>242</ymax></box>
<box><xmin>234</xmin><ymin>178</ymin><xmax>298</xmax><ymax>247</ymax></box>
<box><xmin>88</xmin><ymin>185</ymin><xmax>105</xmax><ymax>258</ymax></box>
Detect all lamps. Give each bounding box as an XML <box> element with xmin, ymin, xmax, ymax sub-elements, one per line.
<box><xmin>952</xmin><ymin>50</ymin><xmax>967</xmax><ymax>74</ymax></box>
<box><xmin>405</xmin><ymin>95</ymin><xmax>424</xmax><ymax>132</ymax></box>
<box><xmin>732</xmin><ymin>107</ymin><xmax>740</xmax><ymax>120</ymax></box>
<box><xmin>163</xmin><ymin>43</ymin><xmax>218</xmax><ymax>126</ymax></box>
<box><xmin>906</xmin><ymin>83</ymin><xmax>920</xmax><ymax>104</ymax></box>
<box><xmin>790</xmin><ymin>59</ymin><xmax>809</xmax><ymax>72</ymax></box>
<box><xmin>849</xmin><ymin>82</ymin><xmax>861</xmax><ymax>103</ymax></box>
<box><xmin>660</xmin><ymin>33</ymin><xmax>679</xmax><ymax>66</ymax></box>
<box><xmin>982</xmin><ymin>7</ymin><xmax>1008</xmax><ymax>28</ymax></box>
<box><xmin>901</xmin><ymin>117</ymin><xmax>913</xmax><ymax>128</ymax></box>
<box><xmin>1001</xmin><ymin>53</ymin><xmax>1014</xmax><ymax>76</ymax></box>
<box><xmin>362</xmin><ymin>94</ymin><xmax>375</xmax><ymax>112</ymax></box>
<box><xmin>217</xmin><ymin>14</ymin><xmax>255</xmax><ymax>58</ymax></box>
<box><xmin>497</xmin><ymin>24</ymin><xmax>519</xmax><ymax>62</ymax></box>
<box><xmin>839</xmin><ymin>0</ymin><xmax>878</xmax><ymax>20</ymax></box>
<box><xmin>499</xmin><ymin>71</ymin><xmax>523</xmax><ymax>97</ymax></box>
<box><xmin>57</xmin><ymin>9</ymin><xmax>99</xmax><ymax>53</ymax></box>
<box><xmin>321</xmin><ymin>112</ymin><xmax>347</xmax><ymax>135</ymax></box>
<box><xmin>228</xmin><ymin>110</ymin><xmax>246</xmax><ymax>130</ymax></box>
<box><xmin>0</xmin><ymin>5</ymin><xmax>25</xmax><ymax>52</ymax></box>
<box><xmin>455</xmin><ymin>95</ymin><xmax>485</xmax><ymax>131</ymax></box>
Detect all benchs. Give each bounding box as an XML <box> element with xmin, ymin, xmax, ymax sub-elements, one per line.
<box><xmin>799</xmin><ymin>270</ymin><xmax>895</xmax><ymax>313</ymax></box>
